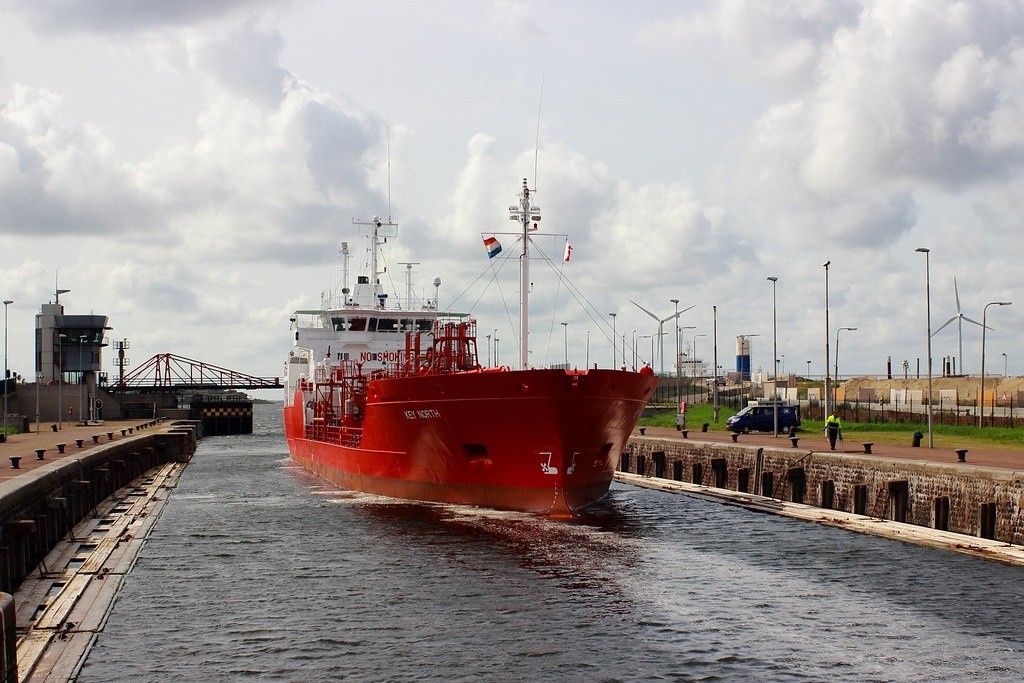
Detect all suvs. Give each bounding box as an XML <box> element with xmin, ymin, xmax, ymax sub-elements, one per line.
<box><xmin>706</xmin><ymin>376</ymin><xmax>726</xmax><ymax>386</ymax></box>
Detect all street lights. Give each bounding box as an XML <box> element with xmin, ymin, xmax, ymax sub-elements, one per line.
<box><xmin>712</xmin><ymin>305</ymin><xmax>720</xmax><ymax>423</ymax></box>
<box><xmin>767</xmin><ymin>277</ymin><xmax>784</xmax><ymax>437</ymax></box>
<box><xmin>36</xmin><ymin>313</ymin><xmax>45</xmax><ymax>433</ymax></box>
<box><xmin>807</xmin><ymin>361</ymin><xmax>811</xmax><ymax>378</ymax></box>
<box><xmin>561</xmin><ymin>322</ymin><xmax>568</xmax><ymax>370</ymax></box>
<box><xmin>80</xmin><ymin>335</ymin><xmax>87</xmax><ymax>422</ymax></box>
<box><xmin>671</xmin><ymin>299</ymin><xmax>680</xmax><ymax>402</ymax></box>
<box><xmin>3</xmin><ymin>301</ymin><xmax>14</xmax><ymax>440</ymax></box>
<box><xmin>608</xmin><ymin>313</ymin><xmax>616</xmax><ymax>371</ymax></box>
<box><xmin>485</xmin><ymin>329</ymin><xmax>499</xmax><ymax>369</ymax></box>
<box><xmin>636</xmin><ymin>333</ymin><xmax>668</xmax><ymax>372</ymax></box>
<box><xmin>693</xmin><ymin>334</ymin><xmax>707</xmax><ymax>404</ymax></box>
<box><xmin>915</xmin><ymin>247</ymin><xmax>932</xmax><ymax>449</ymax></box>
<box><xmin>58</xmin><ymin>334</ymin><xmax>67</xmax><ymax>428</ymax></box>
<box><xmin>822</xmin><ymin>261</ymin><xmax>858</xmax><ymax>438</ymax></box>
<box><xmin>680</xmin><ymin>326</ymin><xmax>696</xmax><ymax>402</ymax></box>
<box><xmin>740</xmin><ymin>335</ymin><xmax>760</xmax><ymax>410</ymax></box>
<box><xmin>979</xmin><ymin>301</ymin><xmax>1008</xmax><ymax>429</ymax></box>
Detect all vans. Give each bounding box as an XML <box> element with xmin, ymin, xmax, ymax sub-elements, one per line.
<box><xmin>727</xmin><ymin>405</ymin><xmax>801</xmax><ymax>434</ymax></box>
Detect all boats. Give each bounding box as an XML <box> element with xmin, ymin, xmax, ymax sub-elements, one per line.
<box><xmin>284</xmin><ymin>75</ymin><xmax>664</xmax><ymax>516</ymax></box>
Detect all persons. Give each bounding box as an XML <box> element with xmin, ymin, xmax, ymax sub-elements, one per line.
<box><xmin>823</xmin><ymin>411</ymin><xmax>843</xmax><ymax>450</ymax></box>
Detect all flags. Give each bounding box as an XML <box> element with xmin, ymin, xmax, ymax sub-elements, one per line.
<box><xmin>564</xmin><ymin>242</ymin><xmax>573</xmax><ymax>262</ymax></box>
<box><xmin>483</xmin><ymin>237</ymin><xmax>502</xmax><ymax>258</ymax></box>
